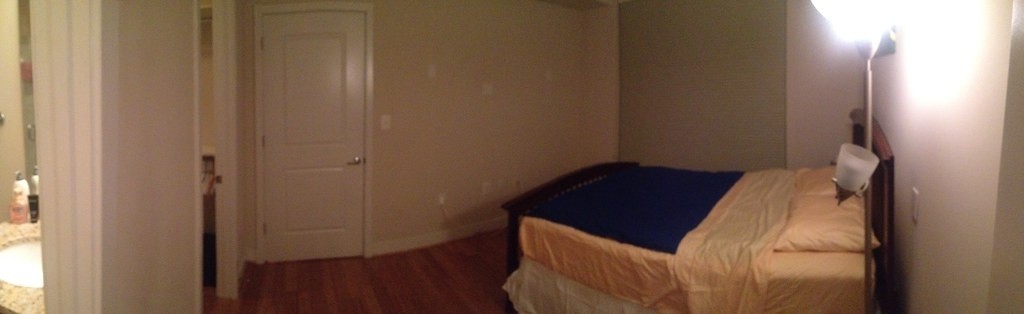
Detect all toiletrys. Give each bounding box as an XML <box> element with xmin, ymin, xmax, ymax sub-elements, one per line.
<box><xmin>8</xmin><ymin>171</ymin><xmax>39</xmax><ymax>224</ymax></box>
<box><xmin>30</xmin><ymin>165</ymin><xmax>40</xmax><ymax>194</ymax></box>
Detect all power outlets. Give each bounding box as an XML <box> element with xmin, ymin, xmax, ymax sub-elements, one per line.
<box><xmin>910</xmin><ymin>187</ymin><xmax>921</xmax><ymax>223</ymax></box>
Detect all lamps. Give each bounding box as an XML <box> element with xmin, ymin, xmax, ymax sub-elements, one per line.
<box><xmin>810</xmin><ymin>0</ymin><xmax>894</xmax><ymax>314</ymax></box>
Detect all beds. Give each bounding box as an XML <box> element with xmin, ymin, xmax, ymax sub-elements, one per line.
<box><xmin>501</xmin><ymin>107</ymin><xmax>896</xmax><ymax>314</ymax></box>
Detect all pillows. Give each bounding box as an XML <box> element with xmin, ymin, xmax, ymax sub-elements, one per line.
<box><xmin>772</xmin><ymin>160</ymin><xmax>881</xmax><ymax>253</ymax></box>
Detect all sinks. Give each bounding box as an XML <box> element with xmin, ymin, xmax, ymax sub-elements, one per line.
<box><xmin>0</xmin><ymin>236</ymin><xmax>45</xmax><ymax>289</ymax></box>
